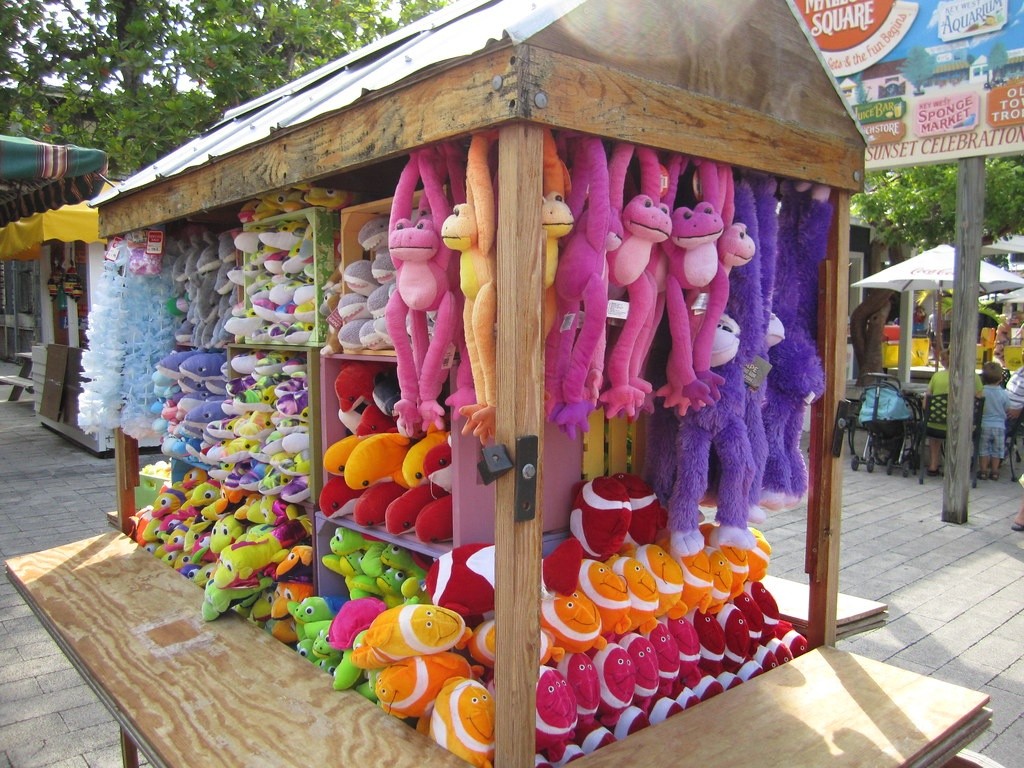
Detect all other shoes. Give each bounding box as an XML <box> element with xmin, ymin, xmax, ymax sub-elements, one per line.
<box><xmin>1011</xmin><ymin>522</ymin><xmax>1024</xmax><ymax>531</ymax></box>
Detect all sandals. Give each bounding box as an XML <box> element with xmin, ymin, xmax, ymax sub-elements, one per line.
<box><xmin>976</xmin><ymin>471</ymin><xmax>988</xmax><ymax>480</ymax></box>
<box><xmin>989</xmin><ymin>471</ymin><xmax>999</xmax><ymax>480</ymax></box>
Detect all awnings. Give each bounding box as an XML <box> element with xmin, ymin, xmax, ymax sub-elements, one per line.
<box><xmin>0</xmin><ymin>135</ymin><xmax>123</xmax><ymax>261</ymax></box>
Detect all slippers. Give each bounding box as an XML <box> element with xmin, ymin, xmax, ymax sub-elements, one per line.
<box><xmin>927</xmin><ymin>468</ymin><xmax>939</xmax><ymax>476</ymax></box>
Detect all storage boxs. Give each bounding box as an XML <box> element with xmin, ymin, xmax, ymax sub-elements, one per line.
<box><xmin>133</xmin><ymin>186</ymin><xmax>586</xmax><ymax>675</ymax></box>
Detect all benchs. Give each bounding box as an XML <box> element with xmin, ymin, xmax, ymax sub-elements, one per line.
<box><xmin>0</xmin><ymin>375</ymin><xmax>34</xmax><ymax>389</ymax></box>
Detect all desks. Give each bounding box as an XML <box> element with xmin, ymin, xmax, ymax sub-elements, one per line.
<box><xmin>0</xmin><ymin>353</ymin><xmax>34</xmax><ymax>402</ymax></box>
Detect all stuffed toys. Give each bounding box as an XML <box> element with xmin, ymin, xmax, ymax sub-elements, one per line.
<box><xmin>47</xmin><ymin>267</ymin><xmax>83</xmax><ymax>303</ymax></box>
<box><xmin>128</xmin><ymin>125</ymin><xmax>831</xmax><ymax>768</ymax></box>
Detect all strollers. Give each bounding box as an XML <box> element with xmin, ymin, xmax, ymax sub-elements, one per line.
<box><xmin>852</xmin><ymin>372</ymin><xmax>925</xmax><ymax>477</ymax></box>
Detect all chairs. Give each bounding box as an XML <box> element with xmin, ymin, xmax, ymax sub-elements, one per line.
<box><xmin>978</xmin><ymin>405</ymin><xmax>1024</xmax><ymax>483</ymax></box>
<box><xmin>914</xmin><ymin>392</ymin><xmax>985</xmax><ymax>488</ymax></box>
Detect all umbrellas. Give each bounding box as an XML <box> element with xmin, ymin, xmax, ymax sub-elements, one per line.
<box><xmin>998</xmin><ymin>287</ymin><xmax>1024</xmax><ymax>303</ymax></box>
<box><xmin>981</xmin><ymin>235</ymin><xmax>1024</xmax><ymax>255</ymax></box>
<box><xmin>849</xmin><ymin>242</ymin><xmax>1024</xmax><ymax>373</ymax></box>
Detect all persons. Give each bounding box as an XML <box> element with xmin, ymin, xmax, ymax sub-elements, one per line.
<box><xmin>926</xmin><ymin>313</ymin><xmax>935</xmax><ymax>360</ymax></box>
<box><xmin>921</xmin><ymin>349</ymin><xmax>983</xmax><ymax>476</ymax></box>
<box><xmin>1005</xmin><ymin>354</ymin><xmax>1024</xmax><ymax>433</ymax></box>
<box><xmin>993</xmin><ymin>314</ymin><xmax>1010</xmax><ymax>368</ymax></box>
<box><xmin>1012</xmin><ymin>323</ymin><xmax>1024</xmax><ymax>352</ymax></box>
<box><xmin>914</xmin><ymin>306</ymin><xmax>925</xmax><ymax>329</ymax></box>
<box><xmin>977</xmin><ymin>361</ymin><xmax>1010</xmax><ymax>480</ymax></box>
<box><xmin>1011</xmin><ymin>473</ymin><xmax>1024</xmax><ymax>531</ymax></box>
